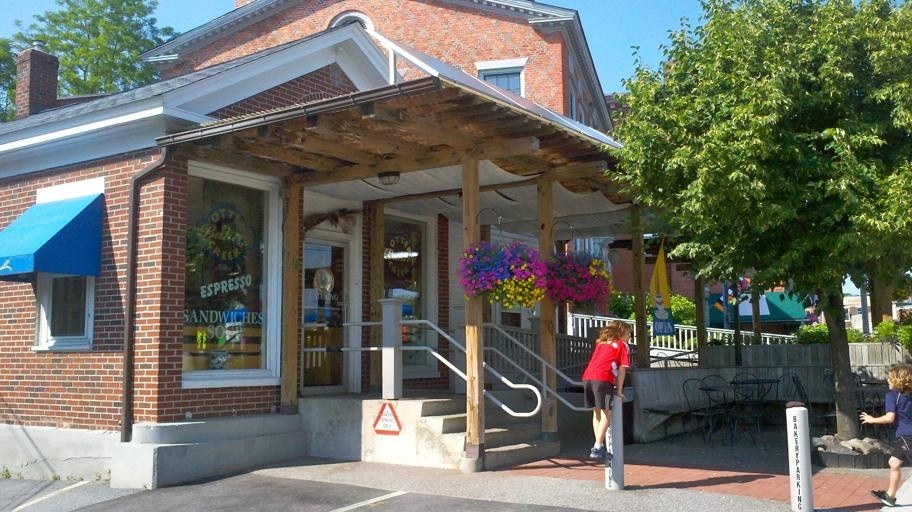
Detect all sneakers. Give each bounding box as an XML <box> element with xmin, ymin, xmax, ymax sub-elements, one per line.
<box><xmin>871</xmin><ymin>489</ymin><xmax>896</xmax><ymax>507</ymax></box>
<box><xmin>590</xmin><ymin>446</ymin><xmax>612</xmax><ymax>458</ymax></box>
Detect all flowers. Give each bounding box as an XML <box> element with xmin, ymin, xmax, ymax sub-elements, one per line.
<box><xmin>459</xmin><ymin>235</ymin><xmax>550</xmax><ymax>314</ymax></box>
<box><xmin>542</xmin><ymin>248</ymin><xmax>611</xmax><ymax>311</ymax></box>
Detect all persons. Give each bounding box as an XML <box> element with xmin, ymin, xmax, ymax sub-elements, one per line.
<box><xmin>582</xmin><ymin>320</ymin><xmax>632</xmax><ymax>459</ymax></box>
<box><xmin>859</xmin><ymin>365</ymin><xmax>912</xmax><ymax>507</ymax></box>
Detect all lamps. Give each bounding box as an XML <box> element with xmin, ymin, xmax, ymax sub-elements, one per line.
<box><xmin>377</xmin><ymin>169</ymin><xmax>403</xmax><ymax>185</ymax></box>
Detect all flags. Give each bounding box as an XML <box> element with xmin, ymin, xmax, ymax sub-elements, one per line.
<box><xmin>649</xmin><ymin>245</ymin><xmax>675</xmax><ymax>337</ymax></box>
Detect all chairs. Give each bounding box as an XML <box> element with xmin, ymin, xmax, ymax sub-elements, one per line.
<box><xmin>638</xmin><ymin>364</ymin><xmax>896</xmax><ymax>453</ymax></box>
